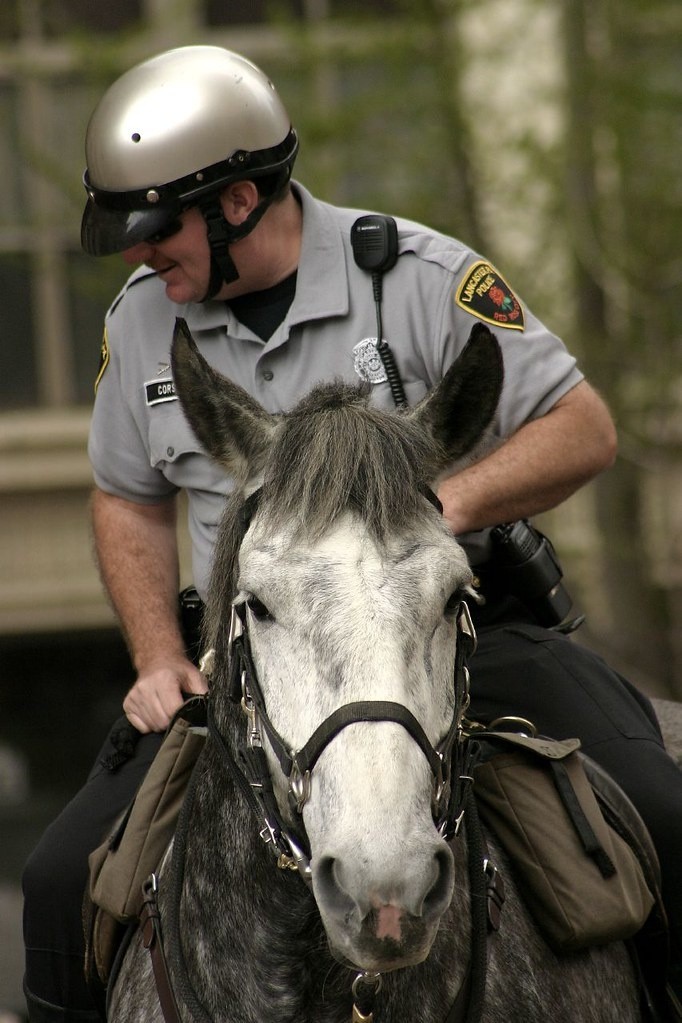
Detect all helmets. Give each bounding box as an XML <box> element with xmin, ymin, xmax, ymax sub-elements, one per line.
<box><xmin>81</xmin><ymin>44</ymin><xmax>299</xmax><ymax>257</ymax></box>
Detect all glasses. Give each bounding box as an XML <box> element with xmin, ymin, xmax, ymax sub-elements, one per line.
<box><xmin>142</xmin><ymin>199</ymin><xmax>195</xmax><ymax>245</ymax></box>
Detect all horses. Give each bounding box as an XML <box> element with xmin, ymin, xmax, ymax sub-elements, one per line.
<box><xmin>99</xmin><ymin>314</ymin><xmax>682</xmax><ymax>1023</ymax></box>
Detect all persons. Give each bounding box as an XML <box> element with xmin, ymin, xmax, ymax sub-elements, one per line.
<box><xmin>24</xmin><ymin>45</ymin><xmax>681</xmax><ymax>1023</ymax></box>
